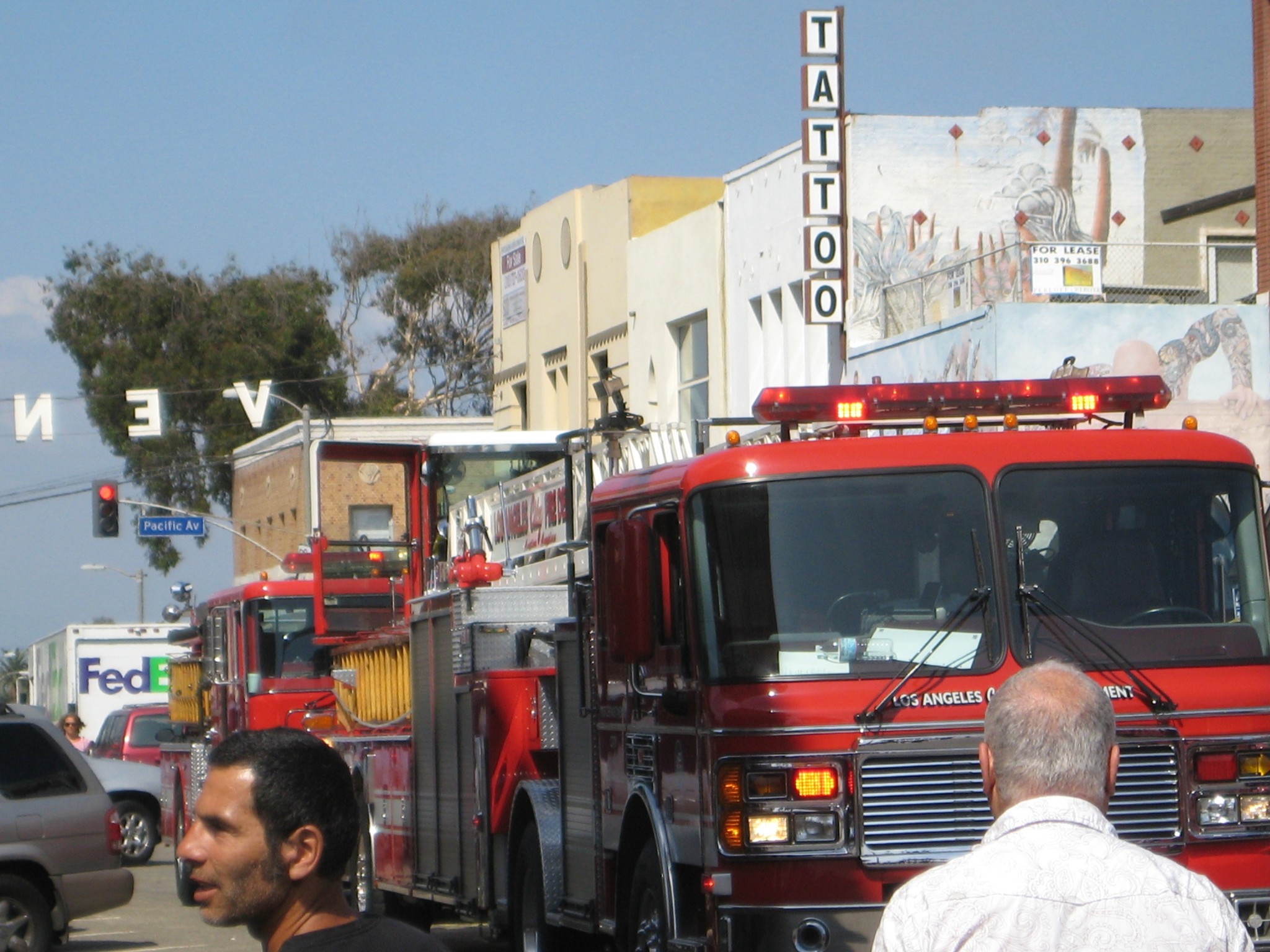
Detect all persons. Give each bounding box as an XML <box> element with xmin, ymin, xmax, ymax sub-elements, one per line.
<box><xmin>871</xmin><ymin>661</ymin><xmax>1258</xmax><ymax>952</ymax></box>
<box><xmin>57</xmin><ymin>713</ymin><xmax>94</xmax><ymax>755</ymax></box>
<box><xmin>177</xmin><ymin>726</ymin><xmax>454</xmax><ymax>952</ymax></box>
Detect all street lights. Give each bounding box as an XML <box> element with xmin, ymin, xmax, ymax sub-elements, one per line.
<box><xmin>222</xmin><ymin>388</ymin><xmax>312</xmax><ymax>535</ymax></box>
<box><xmin>81</xmin><ymin>564</ymin><xmax>147</xmax><ymax>624</ymax></box>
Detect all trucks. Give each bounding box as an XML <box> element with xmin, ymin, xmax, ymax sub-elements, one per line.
<box><xmin>26</xmin><ymin>622</ymin><xmax>192</xmax><ymax>746</ymax></box>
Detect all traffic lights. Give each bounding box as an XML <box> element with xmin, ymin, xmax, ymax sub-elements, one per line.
<box><xmin>92</xmin><ymin>479</ymin><xmax>119</xmax><ymax>538</ymax></box>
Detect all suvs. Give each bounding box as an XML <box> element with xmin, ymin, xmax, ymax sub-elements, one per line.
<box><xmin>86</xmin><ymin>704</ymin><xmax>170</xmax><ymax>767</ymax></box>
<box><xmin>0</xmin><ymin>703</ymin><xmax>136</xmax><ymax>952</ymax></box>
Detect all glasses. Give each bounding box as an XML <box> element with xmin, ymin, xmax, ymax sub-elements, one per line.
<box><xmin>66</xmin><ymin>723</ymin><xmax>76</xmax><ymax>727</ymax></box>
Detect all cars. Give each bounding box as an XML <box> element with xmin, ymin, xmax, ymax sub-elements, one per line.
<box><xmin>74</xmin><ymin>748</ymin><xmax>161</xmax><ymax>868</ymax></box>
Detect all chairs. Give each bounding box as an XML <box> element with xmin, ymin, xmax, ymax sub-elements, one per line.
<box><xmin>258</xmin><ymin>627</ymin><xmax>275</xmax><ymax>678</ymax></box>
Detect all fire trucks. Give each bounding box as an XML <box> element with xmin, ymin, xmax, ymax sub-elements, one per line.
<box><xmin>156</xmin><ymin>550</ymin><xmax>405</xmax><ymax>907</ymax></box>
<box><xmin>284</xmin><ymin>373</ymin><xmax>1269</xmax><ymax>952</ymax></box>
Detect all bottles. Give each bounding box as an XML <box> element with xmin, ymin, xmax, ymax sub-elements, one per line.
<box><xmin>814</xmin><ymin>635</ymin><xmax>892</xmax><ymax>662</ymax></box>
<box><xmin>293</xmin><ymin>656</ymin><xmax>302</xmax><ymax>663</ymax></box>
